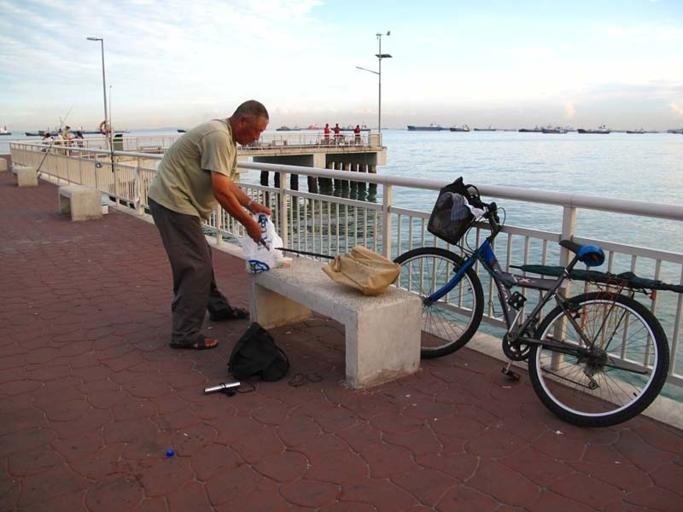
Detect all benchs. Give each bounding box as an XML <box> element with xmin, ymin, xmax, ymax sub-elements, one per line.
<box><xmin>0</xmin><ymin>158</ymin><xmax>7</xmax><ymax>172</ymax></box>
<box><xmin>247</xmin><ymin>256</ymin><xmax>422</xmax><ymax>387</ymax></box>
<box><xmin>10</xmin><ymin>166</ymin><xmax>38</xmax><ymax>186</ymax></box>
<box><xmin>57</xmin><ymin>184</ymin><xmax>102</xmax><ymax>222</ymax></box>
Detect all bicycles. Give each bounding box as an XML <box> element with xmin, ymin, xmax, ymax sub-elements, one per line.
<box><xmin>384</xmin><ymin>184</ymin><xmax>670</xmax><ymax>427</ymax></box>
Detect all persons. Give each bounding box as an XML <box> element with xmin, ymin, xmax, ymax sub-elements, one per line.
<box><xmin>324</xmin><ymin>123</ymin><xmax>361</xmax><ymax>145</ymax></box>
<box><xmin>42</xmin><ymin>125</ymin><xmax>84</xmax><ymax>155</ymax></box>
<box><xmin>147</xmin><ymin>100</ymin><xmax>273</xmax><ymax>350</ymax></box>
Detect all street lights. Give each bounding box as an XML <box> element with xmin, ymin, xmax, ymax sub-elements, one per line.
<box><xmin>87</xmin><ymin>37</ymin><xmax>109</xmax><ymax>138</ymax></box>
<box><xmin>355</xmin><ymin>29</ymin><xmax>392</xmax><ymax>132</ymax></box>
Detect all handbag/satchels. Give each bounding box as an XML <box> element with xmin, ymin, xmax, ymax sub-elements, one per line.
<box><xmin>321</xmin><ymin>245</ymin><xmax>401</xmax><ymax>296</ymax></box>
<box><xmin>440</xmin><ymin>176</ymin><xmax>484</xmax><ymax>210</ymax></box>
<box><xmin>227</xmin><ymin>322</ymin><xmax>289</xmax><ymax>381</ymax></box>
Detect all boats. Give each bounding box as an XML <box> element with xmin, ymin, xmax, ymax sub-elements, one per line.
<box><xmin>331</xmin><ymin>127</ymin><xmax>372</xmax><ymax>131</ymax></box>
<box><xmin>276</xmin><ymin>126</ymin><xmax>301</xmax><ymax>131</ymax></box>
<box><xmin>408</xmin><ymin>126</ymin><xmax>683</xmax><ymax>135</ymax></box>
<box><xmin>25</xmin><ymin>132</ymin><xmax>58</xmax><ymax>136</ymax></box>
<box><xmin>0</xmin><ymin>120</ymin><xmax>12</xmax><ymax>135</ymax></box>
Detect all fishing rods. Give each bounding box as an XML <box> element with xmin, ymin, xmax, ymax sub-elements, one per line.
<box><xmin>36</xmin><ymin>105</ymin><xmax>71</xmax><ymax>172</ymax></box>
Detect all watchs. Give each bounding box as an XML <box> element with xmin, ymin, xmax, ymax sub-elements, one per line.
<box><xmin>246</xmin><ymin>197</ymin><xmax>255</xmax><ymax>209</ymax></box>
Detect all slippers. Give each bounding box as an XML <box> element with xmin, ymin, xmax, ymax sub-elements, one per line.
<box><xmin>210</xmin><ymin>305</ymin><xmax>250</xmax><ymax>321</ymax></box>
<box><xmin>169</xmin><ymin>334</ymin><xmax>219</xmax><ymax>349</ymax></box>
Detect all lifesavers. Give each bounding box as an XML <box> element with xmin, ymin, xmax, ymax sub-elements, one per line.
<box><xmin>100</xmin><ymin>120</ymin><xmax>110</xmax><ymax>135</ymax></box>
